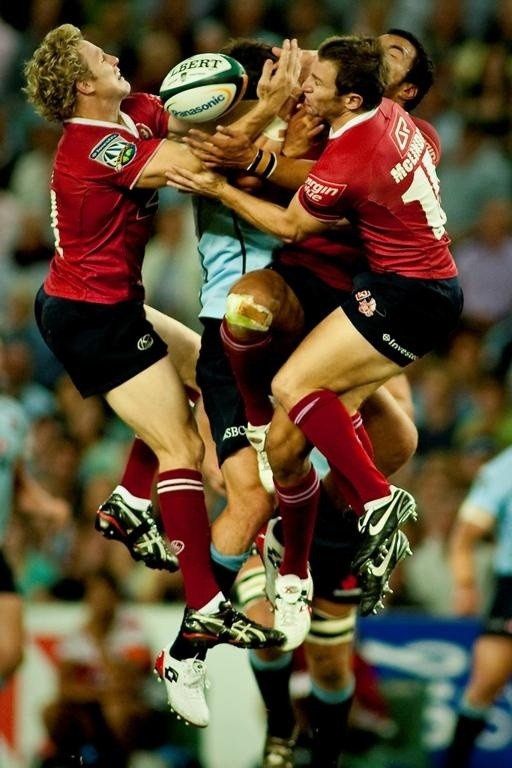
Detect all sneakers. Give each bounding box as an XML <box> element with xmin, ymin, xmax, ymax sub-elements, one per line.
<box><xmin>355</xmin><ymin>530</ymin><xmax>411</xmax><ymax>616</ymax></box>
<box><xmin>179</xmin><ymin>599</ymin><xmax>286</xmax><ymax>649</ymax></box>
<box><xmin>153</xmin><ymin>648</ymin><xmax>212</xmax><ymax>728</ymax></box>
<box><xmin>253</xmin><ymin>515</ymin><xmax>284</xmax><ymax>609</ymax></box>
<box><xmin>94</xmin><ymin>494</ymin><xmax>180</xmax><ymax>573</ymax></box>
<box><xmin>273</xmin><ymin>567</ymin><xmax>314</xmax><ymax>651</ymax></box>
<box><xmin>263</xmin><ymin>724</ymin><xmax>300</xmax><ymax>768</ymax></box>
<box><xmin>351</xmin><ymin>486</ymin><xmax>417</xmax><ymax>575</ymax></box>
<box><xmin>244</xmin><ymin>419</ymin><xmax>276</xmax><ymax>494</ymax></box>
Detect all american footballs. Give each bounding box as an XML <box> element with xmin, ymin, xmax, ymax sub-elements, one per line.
<box><xmin>161</xmin><ymin>54</ymin><xmax>248</xmax><ymax>121</ymax></box>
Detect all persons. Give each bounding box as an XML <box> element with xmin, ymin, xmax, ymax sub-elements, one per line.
<box><xmin>1</xmin><ymin>0</ymin><xmax>512</xmax><ymax>768</ymax></box>
